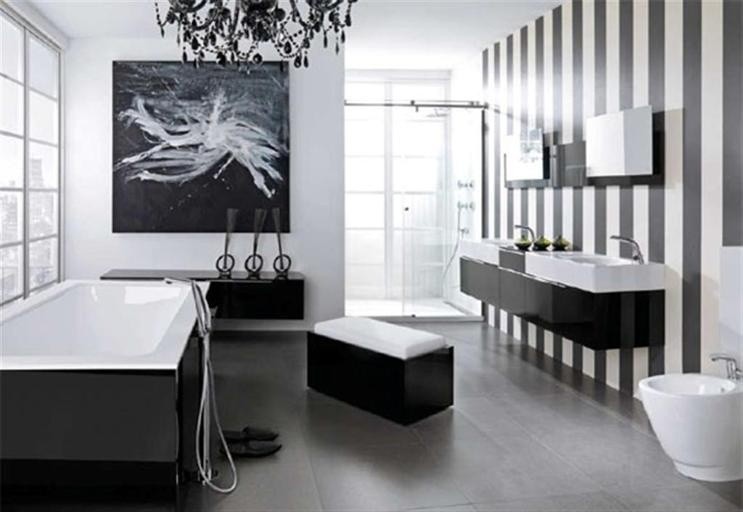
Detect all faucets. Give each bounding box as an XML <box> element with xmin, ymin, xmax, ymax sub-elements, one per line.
<box><xmin>610</xmin><ymin>235</ymin><xmax>644</xmax><ymax>263</ymax></box>
<box><xmin>514</xmin><ymin>224</ymin><xmax>536</xmax><ymax>249</ymax></box>
<box><xmin>709</xmin><ymin>354</ymin><xmax>738</xmax><ymax>376</ymax></box>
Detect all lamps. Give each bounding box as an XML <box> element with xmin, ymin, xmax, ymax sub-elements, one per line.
<box><xmin>152</xmin><ymin>0</ymin><xmax>357</xmax><ymax>76</ymax></box>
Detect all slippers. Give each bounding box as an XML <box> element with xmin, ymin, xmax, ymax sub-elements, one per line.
<box><xmin>218</xmin><ymin>426</ymin><xmax>278</xmax><ymax>441</ymax></box>
<box><xmin>220</xmin><ymin>441</ymin><xmax>282</xmax><ymax>458</ymax></box>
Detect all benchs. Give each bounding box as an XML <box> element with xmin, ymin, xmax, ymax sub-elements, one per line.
<box><xmin>306</xmin><ymin>315</ymin><xmax>454</xmax><ymax>428</ymax></box>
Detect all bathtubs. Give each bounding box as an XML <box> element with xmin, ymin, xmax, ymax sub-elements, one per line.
<box><xmin>2</xmin><ymin>278</ymin><xmax>207</xmax><ymax>373</ymax></box>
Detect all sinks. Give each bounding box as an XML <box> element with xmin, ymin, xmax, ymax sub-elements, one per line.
<box><xmin>460</xmin><ymin>239</ymin><xmax>513</xmax><ymax>265</ymax></box>
<box><xmin>638</xmin><ymin>372</ymin><xmax>743</xmax><ymax>484</ymax></box>
<box><xmin>525</xmin><ymin>250</ymin><xmax>663</xmax><ymax>293</ymax></box>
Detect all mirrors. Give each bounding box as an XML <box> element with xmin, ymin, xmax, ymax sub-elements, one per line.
<box><xmin>505</xmin><ymin>128</ymin><xmax>545</xmax><ymax>181</ymax></box>
<box><xmin>585</xmin><ymin>104</ymin><xmax>654</xmax><ymax>177</ymax></box>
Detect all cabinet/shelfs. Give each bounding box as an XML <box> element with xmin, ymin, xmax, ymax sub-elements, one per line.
<box><xmin>459</xmin><ymin>256</ymin><xmax>666</xmax><ymax>352</ymax></box>
